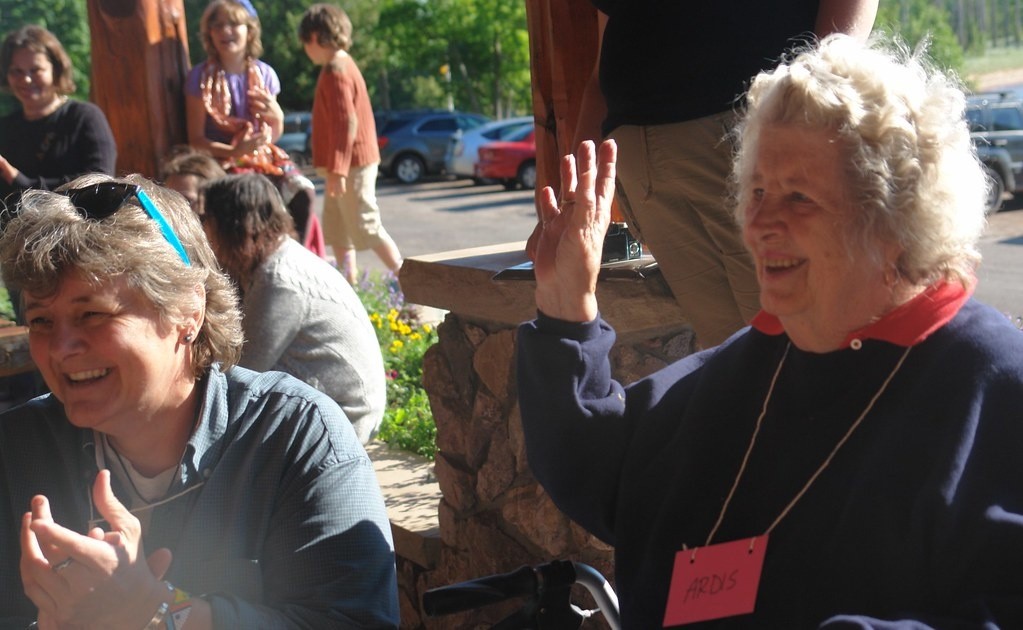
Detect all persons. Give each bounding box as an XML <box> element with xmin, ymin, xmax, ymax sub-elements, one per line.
<box><xmin>203</xmin><ymin>173</ymin><xmax>386</xmax><ymax>446</ymax></box>
<box><xmin>516</xmin><ymin>33</ymin><xmax>1023</xmax><ymax>630</ymax></box>
<box><xmin>184</xmin><ymin>0</ymin><xmax>316</xmax><ymax>247</ymax></box>
<box><xmin>163</xmin><ymin>154</ymin><xmax>229</xmax><ymax>217</ymax></box>
<box><xmin>299</xmin><ymin>4</ymin><xmax>403</xmax><ymax>291</ymax></box>
<box><xmin>525</xmin><ymin>0</ymin><xmax>880</xmax><ymax>350</ymax></box>
<box><xmin>0</xmin><ymin>26</ymin><xmax>117</xmax><ymax>327</ymax></box>
<box><xmin>0</xmin><ymin>172</ymin><xmax>401</xmax><ymax>630</ymax></box>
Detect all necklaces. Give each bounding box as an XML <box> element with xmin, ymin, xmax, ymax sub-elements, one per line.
<box><xmin>105</xmin><ymin>382</ymin><xmax>203</xmax><ymax>504</ymax></box>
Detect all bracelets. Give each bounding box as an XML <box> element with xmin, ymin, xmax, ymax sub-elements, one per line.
<box><xmin>144</xmin><ymin>580</ymin><xmax>193</xmax><ymax>630</ymax></box>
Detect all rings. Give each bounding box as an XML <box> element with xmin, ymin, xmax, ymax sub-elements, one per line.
<box><xmin>561</xmin><ymin>200</ymin><xmax>575</xmax><ymax>204</ymax></box>
<box><xmin>52</xmin><ymin>558</ymin><xmax>73</xmax><ymax>571</ymax></box>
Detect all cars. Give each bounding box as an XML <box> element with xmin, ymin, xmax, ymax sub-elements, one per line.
<box><xmin>274</xmin><ymin>112</ymin><xmax>313</xmax><ymax>166</ymax></box>
<box><xmin>374</xmin><ymin>111</ymin><xmax>495</xmax><ymax>186</ymax></box>
<box><xmin>442</xmin><ymin>116</ymin><xmax>534</xmax><ymax>177</ymax></box>
<box><xmin>472</xmin><ymin>130</ymin><xmax>537</xmax><ymax>190</ymax></box>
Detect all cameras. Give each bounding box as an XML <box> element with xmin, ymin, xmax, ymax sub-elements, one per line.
<box><xmin>601</xmin><ymin>222</ymin><xmax>643</xmax><ymax>265</ymax></box>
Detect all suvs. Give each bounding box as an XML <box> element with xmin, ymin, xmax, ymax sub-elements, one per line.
<box><xmin>965</xmin><ymin>91</ymin><xmax>1023</xmax><ymax>214</ymax></box>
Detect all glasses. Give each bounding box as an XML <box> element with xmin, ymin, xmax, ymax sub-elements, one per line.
<box><xmin>51</xmin><ymin>182</ymin><xmax>191</xmax><ymax>268</ymax></box>
